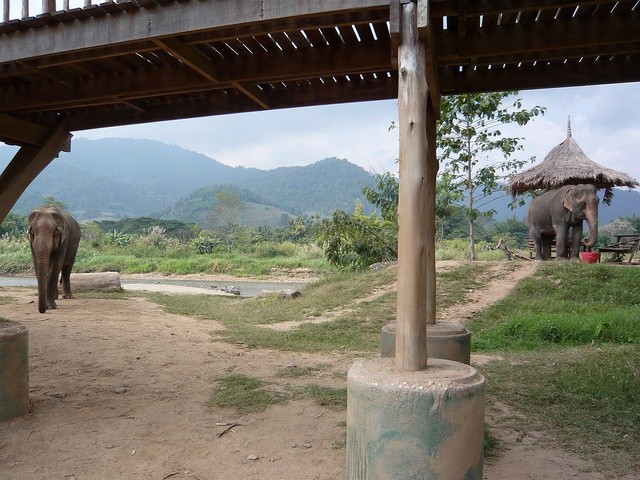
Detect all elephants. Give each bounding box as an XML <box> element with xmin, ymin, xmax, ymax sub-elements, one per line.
<box><xmin>527</xmin><ymin>183</ymin><xmax>600</xmax><ymax>261</ymax></box>
<box><xmin>27</xmin><ymin>205</ymin><xmax>82</xmax><ymax>314</ymax></box>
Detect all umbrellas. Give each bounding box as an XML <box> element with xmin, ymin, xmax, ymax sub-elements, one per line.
<box><xmin>507</xmin><ymin>116</ymin><xmax>640</xmax><ymax>259</ymax></box>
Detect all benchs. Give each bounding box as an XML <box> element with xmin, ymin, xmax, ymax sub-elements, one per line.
<box><xmin>597</xmin><ymin>234</ymin><xmax>640</xmax><ymax>263</ymax></box>
<box><xmin>528</xmin><ymin>236</ymin><xmax>591</xmax><ymax>259</ymax></box>
